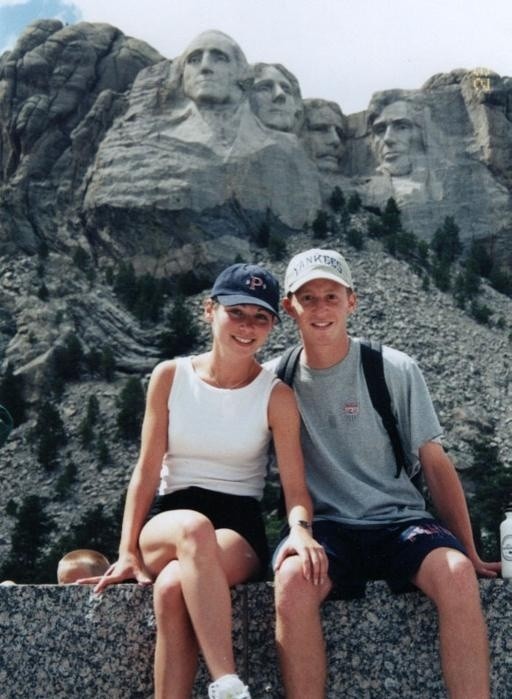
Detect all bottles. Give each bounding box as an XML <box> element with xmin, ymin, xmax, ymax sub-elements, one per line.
<box><xmin>500</xmin><ymin>503</ymin><xmax>512</xmax><ymax>578</ymax></box>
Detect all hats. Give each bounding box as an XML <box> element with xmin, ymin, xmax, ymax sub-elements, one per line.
<box><xmin>284</xmin><ymin>248</ymin><xmax>353</xmax><ymax>299</ymax></box>
<box><xmin>210</xmin><ymin>263</ymin><xmax>282</xmax><ymax>323</ymax></box>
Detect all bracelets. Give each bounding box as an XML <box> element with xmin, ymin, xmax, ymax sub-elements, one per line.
<box><xmin>288</xmin><ymin>520</ymin><xmax>312</xmax><ymax>528</ymax></box>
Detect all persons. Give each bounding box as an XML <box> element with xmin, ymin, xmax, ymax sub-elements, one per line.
<box><xmin>57</xmin><ymin>549</ymin><xmax>109</xmax><ymax>585</ymax></box>
<box><xmin>262</xmin><ymin>249</ymin><xmax>501</xmax><ymax>699</ymax></box>
<box><xmin>75</xmin><ymin>262</ymin><xmax>330</xmax><ymax>698</ymax></box>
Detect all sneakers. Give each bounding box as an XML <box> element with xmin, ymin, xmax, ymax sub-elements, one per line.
<box><xmin>210</xmin><ymin>672</ymin><xmax>250</xmax><ymax>698</ymax></box>
